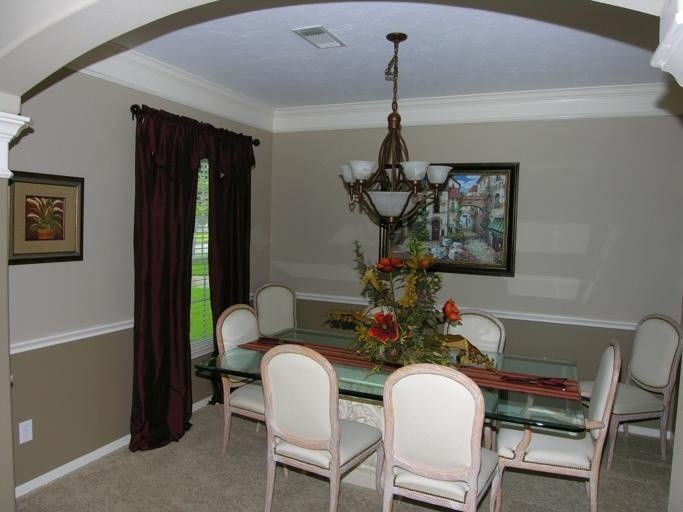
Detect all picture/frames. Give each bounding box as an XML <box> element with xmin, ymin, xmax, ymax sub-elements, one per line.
<box><xmin>8</xmin><ymin>170</ymin><xmax>84</xmax><ymax>265</ymax></box>
<box><xmin>377</xmin><ymin>161</ymin><xmax>520</xmax><ymax>277</ymax></box>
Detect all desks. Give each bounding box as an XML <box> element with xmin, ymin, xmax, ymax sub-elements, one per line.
<box><xmin>193</xmin><ymin>328</ymin><xmax>586</xmax><ymax>492</ymax></box>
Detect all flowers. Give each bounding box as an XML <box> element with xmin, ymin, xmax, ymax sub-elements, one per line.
<box><xmin>320</xmin><ymin>212</ymin><xmax>499</xmax><ymax>380</ymax></box>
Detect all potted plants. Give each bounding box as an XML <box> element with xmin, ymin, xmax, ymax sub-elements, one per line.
<box><xmin>26</xmin><ymin>197</ymin><xmax>64</xmax><ymax>240</ymax></box>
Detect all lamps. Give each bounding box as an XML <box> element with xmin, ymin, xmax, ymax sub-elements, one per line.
<box><xmin>338</xmin><ymin>32</ymin><xmax>454</xmax><ymax>242</ymax></box>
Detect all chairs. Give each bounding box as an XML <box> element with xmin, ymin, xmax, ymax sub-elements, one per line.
<box><xmin>380</xmin><ymin>362</ymin><xmax>503</xmax><ymax>511</ymax></box>
<box><xmin>252</xmin><ymin>283</ymin><xmax>298</xmax><ymax>336</ymax></box>
<box><xmin>215</xmin><ymin>302</ymin><xmax>265</xmax><ymax>457</ymax></box>
<box><xmin>259</xmin><ymin>343</ymin><xmax>386</xmax><ymax>512</ymax></box>
<box><xmin>440</xmin><ymin>308</ymin><xmax>508</xmax><ymax>427</ymax></box>
<box><xmin>491</xmin><ymin>336</ymin><xmax>624</xmax><ymax>512</ymax></box>
<box><xmin>361</xmin><ymin>301</ymin><xmax>407</xmax><ymax>321</ymax></box>
<box><xmin>579</xmin><ymin>310</ymin><xmax>682</xmax><ymax>471</ymax></box>
